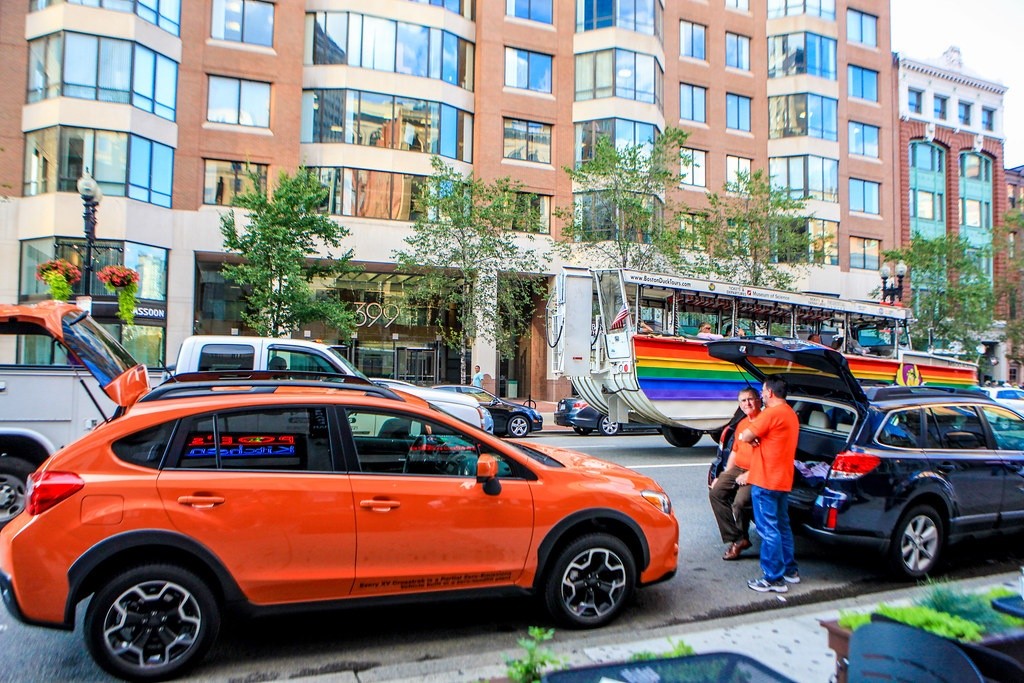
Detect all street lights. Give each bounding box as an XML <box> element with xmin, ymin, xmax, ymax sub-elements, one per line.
<box><xmin>76</xmin><ymin>167</ymin><xmax>104</xmax><ymax>297</ymax></box>
<box><xmin>879</xmin><ymin>261</ymin><xmax>909</xmax><ymax>346</ymax></box>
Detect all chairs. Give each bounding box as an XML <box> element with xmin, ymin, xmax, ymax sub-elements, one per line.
<box><xmin>808</xmin><ymin>411</ymin><xmax>830</xmax><ymax>428</ymax></box>
<box><xmin>836</xmin><ymin>424</ymin><xmax>853</xmax><ymax>432</ymax></box>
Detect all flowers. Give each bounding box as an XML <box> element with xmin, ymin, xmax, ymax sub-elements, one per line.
<box><xmin>97</xmin><ymin>263</ymin><xmax>139</xmax><ymax>284</ymax></box>
<box><xmin>35</xmin><ymin>258</ymin><xmax>81</xmax><ymax>285</ymax></box>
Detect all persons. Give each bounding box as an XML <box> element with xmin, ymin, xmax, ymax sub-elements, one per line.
<box><xmin>709</xmin><ymin>388</ymin><xmax>761</xmax><ymax>561</ymax></box>
<box><xmin>847</xmin><ymin>328</ymin><xmax>871</xmax><ymax>354</ymax></box>
<box><xmin>470</xmin><ymin>365</ymin><xmax>484</xmax><ymax>388</ymax></box>
<box><xmin>632</xmin><ymin>314</ymin><xmax>653</xmax><ymax>333</ymax></box>
<box><xmin>984</xmin><ymin>380</ymin><xmax>1024</xmax><ymax>390</ymax></box>
<box><xmin>697</xmin><ymin>322</ymin><xmax>724</xmax><ymax>339</ymax></box>
<box><xmin>723</xmin><ymin>324</ymin><xmax>744</xmax><ymax>338</ymax></box>
<box><xmin>742</xmin><ymin>376</ymin><xmax>801</xmax><ymax>592</ymax></box>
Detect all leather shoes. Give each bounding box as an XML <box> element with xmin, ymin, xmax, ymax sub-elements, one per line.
<box><xmin>723</xmin><ymin>539</ymin><xmax>752</xmax><ymax>560</ymax></box>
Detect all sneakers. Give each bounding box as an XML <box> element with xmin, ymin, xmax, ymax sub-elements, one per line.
<box><xmin>747</xmin><ymin>576</ymin><xmax>788</xmax><ymax>593</ymax></box>
<box><xmin>763</xmin><ymin>568</ymin><xmax>800</xmax><ymax>583</ymax></box>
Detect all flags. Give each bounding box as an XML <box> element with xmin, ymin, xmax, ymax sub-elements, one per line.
<box><xmin>609</xmin><ymin>305</ymin><xmax>628</xmax><ymax>330</ymax></box>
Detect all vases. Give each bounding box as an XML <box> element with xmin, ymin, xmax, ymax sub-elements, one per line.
<box><xmin>65</xmin><ymin>274</ymin><xmax>72</xmax><ymax>283</ymax></box>
<box><xmin>110</xmin><ymin>277</ymin><xmax>131</xmax><ymax>287</ymax></box>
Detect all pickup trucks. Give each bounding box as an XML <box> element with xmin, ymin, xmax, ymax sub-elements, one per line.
<box><xmin>0</xmin><ymin>336</ymin><xmax>496</xmax><ymax>532</ymax></box>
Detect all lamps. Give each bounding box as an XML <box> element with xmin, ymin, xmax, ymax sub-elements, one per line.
<box><xmin>976</xmin><ymin>344</ymin><xmax>986</xmax><ymax>364</ymax></box>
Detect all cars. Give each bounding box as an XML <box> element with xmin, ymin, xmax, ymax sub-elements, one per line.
<box><xmin>554</xmin><ymin>396</ymin><xmax>664</xmax><ymax>436</ymax></box>
<box><xmin>427</xmin><ymin>384</ymin><xmax>545</xmax><ymax>438</ymax></box>
<box><xmin>980</xmin><ymin>386</ymin><xmax>1024</xmax><ymax>431</ymax></box>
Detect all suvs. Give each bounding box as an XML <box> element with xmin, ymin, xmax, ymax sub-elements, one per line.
<box><xmin>695</xmin><ymin>333</ymin><xmax>1024</xmax><ymax>582</ymax></box>
<box><xmin>0</xmin><ymin>299</ymin><xmax>680</xmax><ymax>683</ymax></box>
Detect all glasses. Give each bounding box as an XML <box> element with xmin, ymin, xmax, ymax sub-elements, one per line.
<box><xmin>706</xmin><ymin>327</ymin><xmax>711</xmax><ymax>330</ymax></box>
